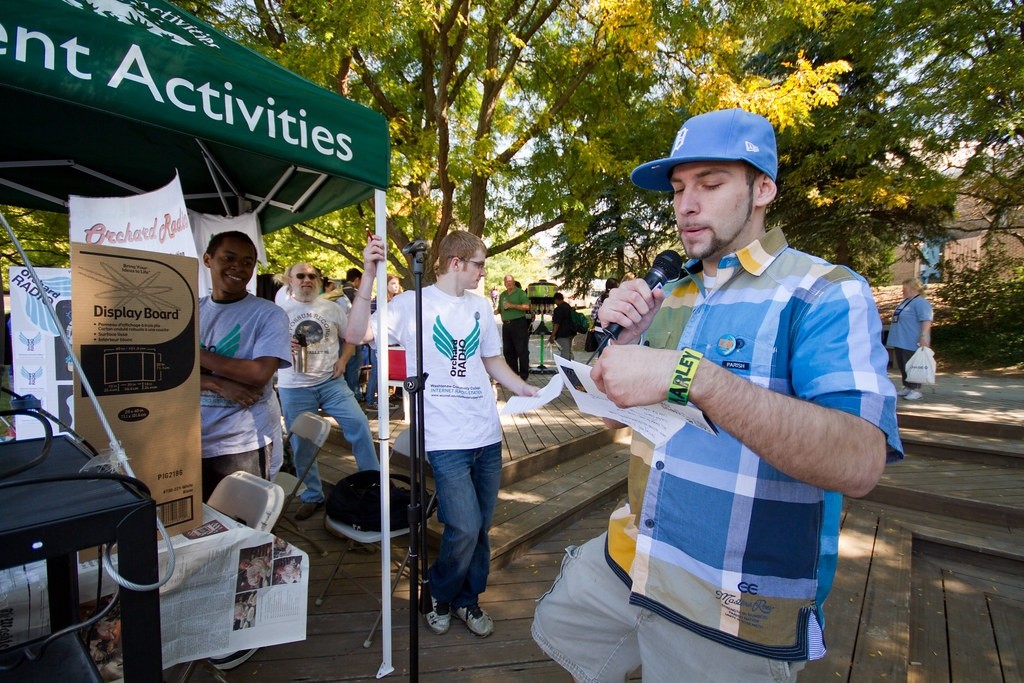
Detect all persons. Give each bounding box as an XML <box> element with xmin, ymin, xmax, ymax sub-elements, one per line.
<box><xmin>344</xmin><ymin>230</ymin><xmax>541</xmax><ymax>637</ymax></box>
<box><xmin>490</xmin><ymin>272</ymin><xmax>637</xmax><ymax>387</ymax></box>
<box><xmin>233</xmin><ymin>538</ymin><xmax>305</xmax><ymax>628</ymax></box>
<box><xmin>531</xmin><ymin>109</ymin><xmax>905</xmax><ymax>683</ymax></box>
<box><xmin>198</xmin><ymin>231</ymin><xmax>292</xmax><ymax>509</ymax></box>
<box><xmin>273</xmin><ymin>262</ymin><xmax>401</xmax><ymax>520</ymax></box>
<box><xmin>887</xmin><ymin>278</ymin><xmax>934</xmax><ymax>401</ymax></box>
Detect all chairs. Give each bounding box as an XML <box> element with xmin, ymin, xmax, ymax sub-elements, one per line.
<box><xmin>173</xmin><ymin>471</ymin><xmax>285</xmax><ymax>683</ymax></box>
<box><xmin>316</xmin><ymin>424</ymin><xmax>437</xmax><ymax>650</ymax></box>
<box><xmin>271</xmin><ymin>411</ymin><xmax>331</xmax><ymax>558</ymax></box>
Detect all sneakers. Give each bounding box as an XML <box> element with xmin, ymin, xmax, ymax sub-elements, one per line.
<box><xmin>451</xmin><ymin>603</ymin><xmax>494</xmax><ymax>636</ymax></box>
<box><xmin>897</xmin><ymin>386</ymin><xmax>912</xmax><ymax>396</ymax></box>
<box><xmin>903</xmin><ymin>390</ymin><xmax>923</xmax><ymax>400</ymax></box>
<box><xmin>425</xmin><ymin>595</ymin><xmax>451</xmax><ymax>634</ymax></box>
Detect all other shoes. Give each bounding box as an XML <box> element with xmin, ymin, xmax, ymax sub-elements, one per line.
<box><xmin>295</xmin><ymin>502</ymin><xmax>323</xmax><ymax>521</ymax></box>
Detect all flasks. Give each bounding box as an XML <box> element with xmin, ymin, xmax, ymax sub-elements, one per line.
<box><xmin>291</xmin><ymin>333</ymin><xmax>308</xmax><ymax>373</ymax></box>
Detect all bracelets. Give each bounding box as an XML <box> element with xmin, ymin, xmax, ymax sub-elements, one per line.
<box><xmin>357</xmin><ymin>293</ymin><xmax>371</xmax><ymax>301</ymax></box>
<box><xmin>668</xmin><ymin>348</ymin><xmax>703</xmax><ymax>408</ymax></box>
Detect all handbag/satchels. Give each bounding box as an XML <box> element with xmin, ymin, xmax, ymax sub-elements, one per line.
<box><xmin>569</xmin><ymin>303</ymin><xmax>589</xmax><ymax>334</ymax></box>
<box><xmin>904</xmin><ymin>345</ymin><xmax>937</xmax><ymax>385</ymax></box>
<box><xmin>584</xmin><ymin>332</ymin><xmax>599</xmax><ymax>352</ymax></box>
<box><xmin>326</xmin><ymin>470</ymin><xmax>429</xmax><ymax>532</ymax></box>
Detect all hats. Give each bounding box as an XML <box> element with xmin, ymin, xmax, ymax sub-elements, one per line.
<box><xmin>630</xmin><ymin>109</ymin><xmax>779</xmax><ymax>192</ymax></box>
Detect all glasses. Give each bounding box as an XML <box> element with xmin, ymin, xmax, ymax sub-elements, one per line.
<box><xmin>449</xmin><ymin>256</ymin><xmax>484</xmax><ymax>270</ymax></box>
<box><xmin>290</xmin><ymin>273</ymin><xmax>317</xmax><ymax>280</ymax></box>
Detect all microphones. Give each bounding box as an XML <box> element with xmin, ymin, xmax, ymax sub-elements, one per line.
<box><xmin>586</xmin><ymin>250</ymin><xmax>683</xmax><ymax>366</ymax></box>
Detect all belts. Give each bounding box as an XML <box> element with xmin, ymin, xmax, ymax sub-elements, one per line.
<box><xmin>503</xmin><ymin>316</ymin><xmax>525</xmax><ymax>323</ymax></box>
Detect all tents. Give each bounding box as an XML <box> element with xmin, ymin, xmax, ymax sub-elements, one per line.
<box><xmin>0</xmin><ymin>0</ymin><xmax>396</xmax><ymax>679</ymax></box>
